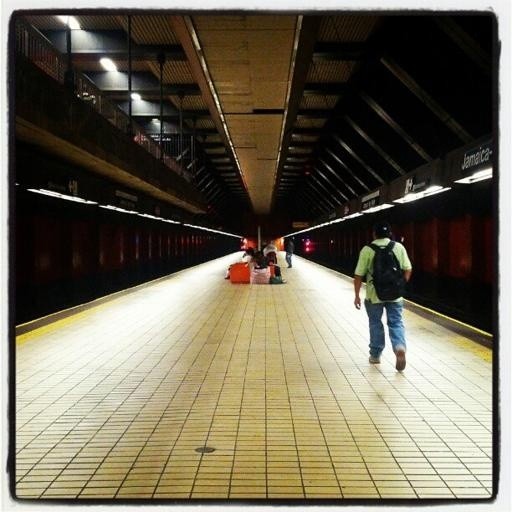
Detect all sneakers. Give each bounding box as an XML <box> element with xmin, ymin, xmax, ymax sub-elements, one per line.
<box><xmin>368</xmin><ymin>354</ymin><xmax>382</xmax><ymax>364</ymax></box>
<box><xmin>393</xmin><ymin>344</ymin><xmax>408</xmax><ymax>371</ymax></box>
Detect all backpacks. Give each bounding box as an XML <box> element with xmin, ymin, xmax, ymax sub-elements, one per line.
<box><xmin>364</xmin><ymin>240</ymin><xmax>408</xmax><ymax>302</ymax></box>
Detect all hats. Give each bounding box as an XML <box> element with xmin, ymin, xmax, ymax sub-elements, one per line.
<box><xmin>370</xmin><ymin>220</ymin><xmax>393</xmax><ymax>238</ymax></box>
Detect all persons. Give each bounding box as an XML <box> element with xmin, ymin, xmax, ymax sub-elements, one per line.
<box><xmin>224</xmin><ymin>239</ymin><xmax>287</xmax><ymax>284</ymax></box>
<box><xmin>283</xmin><ymin>236</ymin><xmax>295</xmax><ymax>268</ymax></box>
<box><xmin>352</xmin><ymin>219</ymin><xmax>413</xmax><ymax>370</ymax></box>
